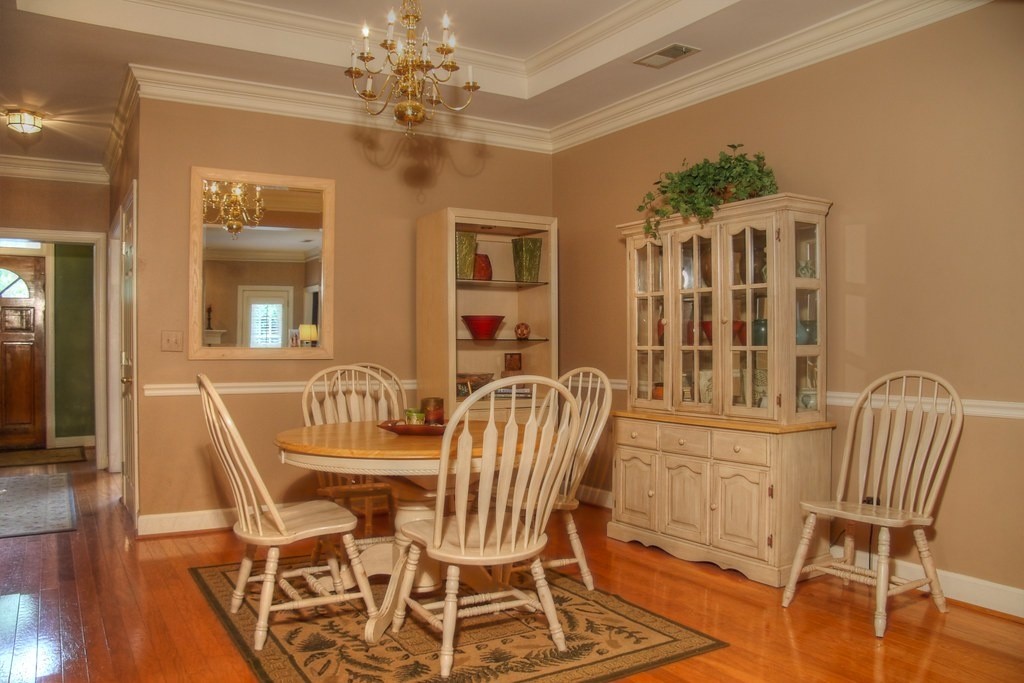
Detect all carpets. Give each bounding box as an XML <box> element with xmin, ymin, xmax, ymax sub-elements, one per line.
<box><xmin>188</xmin><ymin>553</ymin><xmax>730</xmax><ymax>683</ymax></box>
<box><xmin>0</xmin><ymin>471</ymin><xmax>78</xmax><ymax>538</ymax></box>
<box><xmin>0</xmin><ymin>445</ymin><xmax>89</xmax><ymax>468</ymax></box>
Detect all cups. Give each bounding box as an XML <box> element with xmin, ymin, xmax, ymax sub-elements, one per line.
<box><xmin>419</xmin><ymin>396</ymin><xmax>444</xmax><ymax>425</ymax></box>
<box><xmin>404</xmin><ymin>408</ymin><xmax>425</xmax><ymax>425</ymax></box>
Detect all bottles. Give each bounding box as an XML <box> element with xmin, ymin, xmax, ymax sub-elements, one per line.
<box><xmin>797</xmin><ymin>304</ymin><xmax>809</xmax><ymax>345</ymax></box>
<box><xmin>795</xmin><ymin>259</ymin><xmax>815</xmax><ymax>278</ymax></box>
<box><xmin>702</xmin><ymin>245</ymin><xmax>768</xmax><ymax>285</ymax></box>
<box><xmin>683</xmin><ymin>374</ymin><xmax>690</xmax><ymax>401</ymax></box>
<box><xmin>652</xmin><ymin>382</ymin><xmax>663</xmax><ymax>399</ymax></box>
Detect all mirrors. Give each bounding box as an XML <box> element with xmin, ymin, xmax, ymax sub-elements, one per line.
<box><xmin>188</xmin><ymin>164</ymin><xmax>334</xmax><ymax>359</ymax></box>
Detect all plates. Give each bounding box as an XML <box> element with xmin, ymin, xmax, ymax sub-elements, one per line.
<box><xmin>377</xmin><ymin>420</ymin><xmax>465</xmax><ymax>435</ymax></box>
<box><xmin>742</xmin><ymin>370</ymin><xmax>768</xmax><ymax>406</ymax></box>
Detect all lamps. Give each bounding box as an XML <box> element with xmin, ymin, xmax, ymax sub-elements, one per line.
<box><xmin>203</xmin><ymin>180</ymin><xmax>268</xmax><ymax>238</ymax></box>
<box><xmin>7</xmin><ymin>110</ymin><xmax>46</xmax><ymax>133</ymax></box>
<box><xmin>298</xmin><ymin>323</ymin><xmax>317</xmax><ymax>346</ymax></box>
<box><xmin>344</xmin><ymin>1</ymin><xmax>479</xmax><ymax>134</ymax></box>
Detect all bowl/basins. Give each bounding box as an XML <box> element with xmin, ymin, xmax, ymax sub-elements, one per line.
<box><xmin>656</xmin><ymin>318</ymin><xmax>769</xmax><ymax>346</ymax></box>
<box><xmin>460</xmin><ymin>314</ymin><xmax>505</xmax><ymax>339</ymax></box>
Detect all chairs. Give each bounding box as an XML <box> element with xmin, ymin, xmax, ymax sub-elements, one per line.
<box><xmin>781</xmin><ymin>372</ymin><xmax>962</xmax><ymax>639</ymax></box>
<box><xmin>196</xmin><ymin>361</ymin><xmax>614</xmax><ymax>677</ymax></box>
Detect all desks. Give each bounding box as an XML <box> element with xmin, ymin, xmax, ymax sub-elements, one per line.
<box><xmin>274</xmin><ymin>420</ymin><xmax>559</xmax><ymax>613</ymax></box>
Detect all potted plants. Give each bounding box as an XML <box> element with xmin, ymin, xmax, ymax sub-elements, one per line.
<box><xmin>638</xmin><ymin>143</ymin><xmax>779</xmax><ymax>240</ymax></box>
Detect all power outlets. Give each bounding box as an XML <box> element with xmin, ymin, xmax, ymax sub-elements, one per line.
<box><xmin>867</xmin><ymin>498</ymin><xmax>879</xmax><ymax>506</ymax></box>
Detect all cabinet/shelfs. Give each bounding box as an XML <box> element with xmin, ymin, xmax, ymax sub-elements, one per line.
<box><xmin>608</xmin><ymin>191</ymin><xmax>830</xmax><ymax>587</ymax></box>
<box><xmin>415</xmin><ymin>207</ymin><xmax>558</xmax><ymax>515</ymax></box>
